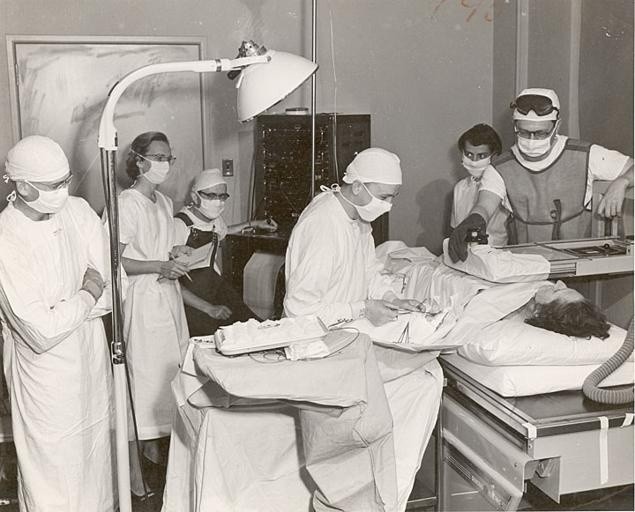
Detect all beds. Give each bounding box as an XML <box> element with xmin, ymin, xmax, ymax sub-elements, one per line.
<box><xmin>378</xmin><ymin>244</ymin><xmax>634</xmax><ymax>508</ymax></box>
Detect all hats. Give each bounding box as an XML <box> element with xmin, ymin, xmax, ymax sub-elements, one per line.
<box><xmin>342</xmin><ymin>147</ymin><xmax>403</xmax><ymax>186</ymax></box>
<box><xmin>3</xmin><ymin>135</ymin><xmax>71</xmax><ymax>184</ymax></box>
<box><xmin>512</xmin><ymin>87</ymin><xmax>561</xmax><ymax>122</ymax></box>
<box><xmin>191</xmin><ymin>168</ymin><xmax>228</xmax><ymax>198</ymax></box>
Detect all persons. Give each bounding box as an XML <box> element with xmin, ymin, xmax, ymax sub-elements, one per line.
<box><xmin>281</xmin><ymin>147</ymin><xmax>429</xmax><ymax>327</ymax></box>
<box><xmin>101</xmin><ymin>130</ymin><xmax>195</xmax><ymax>499</ymax></box>
<box><xmin>447</xmin><ymin>88</ymin><xmax>634</xmax><ymax>263</ymax></box>
<box><xmin>0</xmin><ymin>135</ymin><xmax>130</xmax><ymax>512</ymax></box>
<box><xmin>173</xmin><ymin>167</ymin><xmax>280</xmax><ymax>337</ymax></box>
<box><xmin>501</xmin><ymin>279</ymin><xmax>612</xmax><ymax>340</ymax></box>
<box><xmin>446</xmin><ymin>124</ymin><xmax>516</xmax><ymax>248</ymax></box>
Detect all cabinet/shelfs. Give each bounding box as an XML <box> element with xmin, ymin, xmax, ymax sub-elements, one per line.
<box><xmin>255</xmin><ymin>114</ymin><xmax>371</xmax><ymax>229</ymax></box>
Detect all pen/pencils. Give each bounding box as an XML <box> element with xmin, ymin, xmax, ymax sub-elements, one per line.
<box><xmin>169</xmin><ymin>252</ymin><xmax>193</xmax><ymax>282</ymax></box>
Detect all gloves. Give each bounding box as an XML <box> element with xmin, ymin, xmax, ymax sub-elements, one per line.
<box><xmin>446</xmin><ymin>212</ymin><xmax>488</xmax><ymax>263</ymax></box>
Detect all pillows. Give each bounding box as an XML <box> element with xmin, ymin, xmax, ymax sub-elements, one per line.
<box><xmin>458</xmin><ymin>322</ymin><xmax>634</xmax><ymax>367</ymax></box>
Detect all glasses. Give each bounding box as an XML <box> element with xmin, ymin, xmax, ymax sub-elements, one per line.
<box><xmin>142</xmin><ymin>153</ymin><xmax>176</xmax><ymax>165</ymax></box>
<box><xmin>37</xmin><ymin>173</ymin><xmax>74</xmax><ymax>191</ymax></box>
<box><xmin>512</xmin><ymin>126</ymin><xmax>554</xmax><ymax>137</ymax></box>
<box><xmin>198</xmin><ymin>190</ymin><xmax>230</xmax><ymax>201</ymax></box>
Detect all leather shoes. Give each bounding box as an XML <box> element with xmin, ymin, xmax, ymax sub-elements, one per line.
<box><xmin>131</xmin><ymin>487</ymin><xmax>159</xmax><ymax>511</ymax></box>
<box><xmin>141</xmin><ymin>452</ymin><xmax>166</xmax><ymax>478</ymax></box>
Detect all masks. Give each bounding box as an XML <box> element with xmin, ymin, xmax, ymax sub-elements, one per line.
<box><xmin>17</xmin><ymin>180</ymin><xmax>69</xmax><ymax>214</ymax></box>
<box><xmin>460</xmin><ymin>153</ymin><xmax>495</xmax><ymax>179</ymax></box>
<box><xmin>514</xmin><ymin>121</ymin><xmax>558</xmax><ymax>158</ymax></box>
<box><xmin>192</xmin><ymin>190</ymin><xmax>225</xmax><ymax>220</ymax></box>
<box><xmin>135</xmin><ymin>154</ymin><xmax>170</xmax><ymax>185</ymax></box>
<box><xmin>350</xmin><ymin>178</ymin><xmax>393</xmax><ymax>223</ymax></box>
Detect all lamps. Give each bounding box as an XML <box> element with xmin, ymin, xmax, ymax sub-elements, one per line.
<box><xmin>98</xmin><ymin>40</ymin><xmax>318</xmax><ymax>511</ymax></box>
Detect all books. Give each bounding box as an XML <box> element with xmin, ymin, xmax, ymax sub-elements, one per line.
<box><xmin>156</xmin><ymin>242</ymin><xmax>215</xmax><ymax>283</ymax></box>
